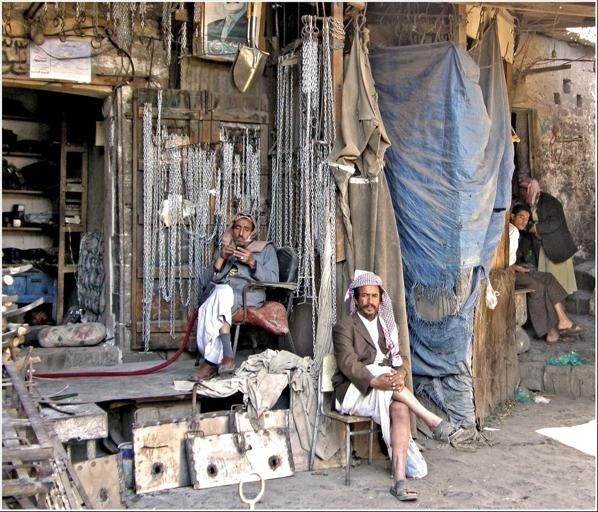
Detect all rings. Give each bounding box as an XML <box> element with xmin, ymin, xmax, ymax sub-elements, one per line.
<box><xmin>240</xmin><ymin>256</ymin><xmax>243</xmax><ymax>260</ymax></box>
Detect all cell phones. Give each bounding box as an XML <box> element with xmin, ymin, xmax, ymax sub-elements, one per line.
<box><xmin>237</xmin><ymin>245</ymin><xmax>245</xmax><ymax>261</ymax></box>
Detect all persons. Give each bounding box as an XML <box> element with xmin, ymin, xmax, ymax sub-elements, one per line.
<box><xmin>187</xmin><ymin>212</ymin><xmax>281</xmax><ymax>384</ymax></box>
<box><xmin>509</xmin><ymin>203</ymin><xmax>589</xmax><ymax>344</ymax></box>
<box><xmin>515</xmin><ymin>176</ymin><xmax>581</xmax><ymax>329</ymax></box>
<box><xmin>331</xmin><ymin>270</ymin><xmax>475</xmax><ymax>501</ymax></box>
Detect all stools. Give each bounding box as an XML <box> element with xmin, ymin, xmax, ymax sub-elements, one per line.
<box><xmin>511</xmin><ymin>285</ymin><xmax>535</xmax><ymax>353</ymax></box>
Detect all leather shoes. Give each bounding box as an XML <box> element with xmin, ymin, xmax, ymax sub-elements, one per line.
<box><xmin>218</xmin><ymin>357</ymin><xmax>237</xmax><ymax>374</ymax></box>
<box><xmin>188</xmin><ymin>362</ymin><xmax>218</xmax><ymax>383</ymax></box>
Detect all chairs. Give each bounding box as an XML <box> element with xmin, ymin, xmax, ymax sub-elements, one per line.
<box><xmin>195</xmin><ymin>246</ymin><xmax>299</xmax><ymax>366</ymax></box>
<box><xmin>307</xmin><ymin>354</ymin><xmax>375</xmax><ymax>486</ymax></box>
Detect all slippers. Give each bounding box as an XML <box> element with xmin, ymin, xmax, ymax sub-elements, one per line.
<box><xmin>558</xmin><ymin>323</ymin><xmax>586</xmax><ymax>335</ymax></box>
<box><xmin>547</xmin><ymin>334</ymin><xmax>575</xmax><ymax>344</ymax></box>
<box><xmin>429</xmin><ymin>420</ymin><xmax>461</xmax><ymax>441</ymax></box>
<box><xmin>389</xmin><ymin>478</ymin><xmax>419</xmax><ymax>501</ymax></box>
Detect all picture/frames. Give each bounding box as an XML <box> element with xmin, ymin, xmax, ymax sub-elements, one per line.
<box><xmin>193</xmin><ymin>1</ymin><xmax>269</xmax><ymax>65</ymax></box>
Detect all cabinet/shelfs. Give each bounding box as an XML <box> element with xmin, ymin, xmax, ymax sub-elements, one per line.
<box><xmin>2</xmin><ymin>73</ymin><xmax>119</xmax><ymax>324</ymax></box>
